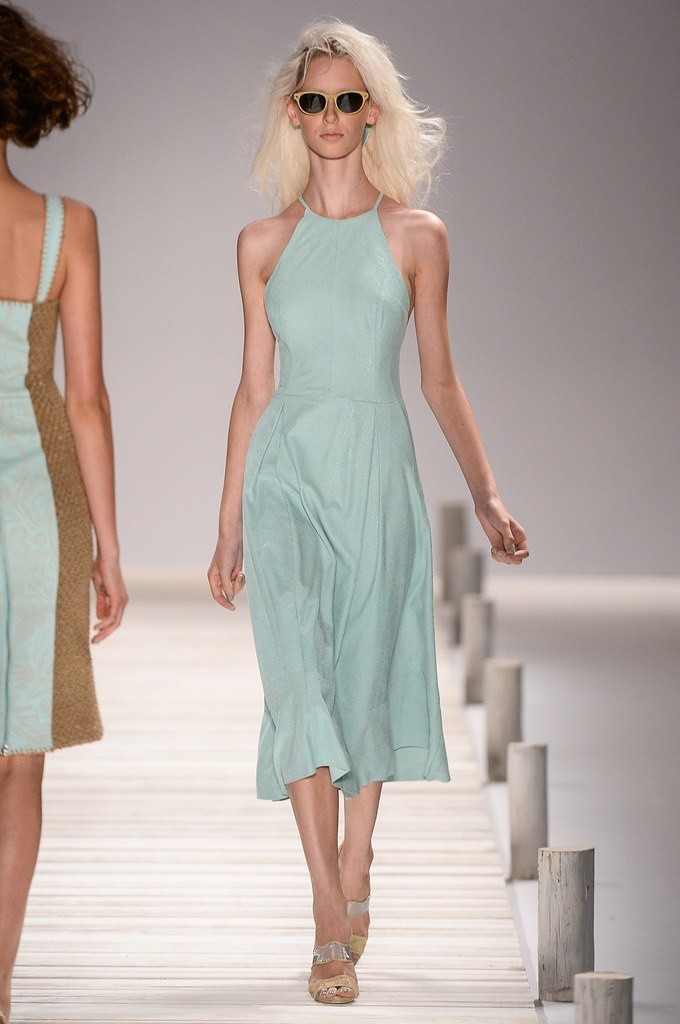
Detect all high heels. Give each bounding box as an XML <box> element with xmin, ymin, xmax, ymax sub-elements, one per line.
<box><xmin>307</xmin><ymin>940</ymin><xmax>360</xmax><ymax>1004</ymax></box>
<box><xmin>338</xmin><ymin>838</ymin><xmax>371</xmax><ymax>966</ymax></box>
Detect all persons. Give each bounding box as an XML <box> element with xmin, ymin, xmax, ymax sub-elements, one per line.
<box><xmin>207</xmin><ymin>21</ymin><xmax>530</xmax><ymax>1004</ymax></box>
<box><xmin>0</xmin><ymin>0</ymin><xmax>130</xmax><ymax>1023</ymax></box>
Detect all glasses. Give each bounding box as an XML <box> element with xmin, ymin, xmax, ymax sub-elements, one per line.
<box><xmin>293</xmin><ymin>90</ymin><xmax>369</xmax><ymax>115</ymax></box>
